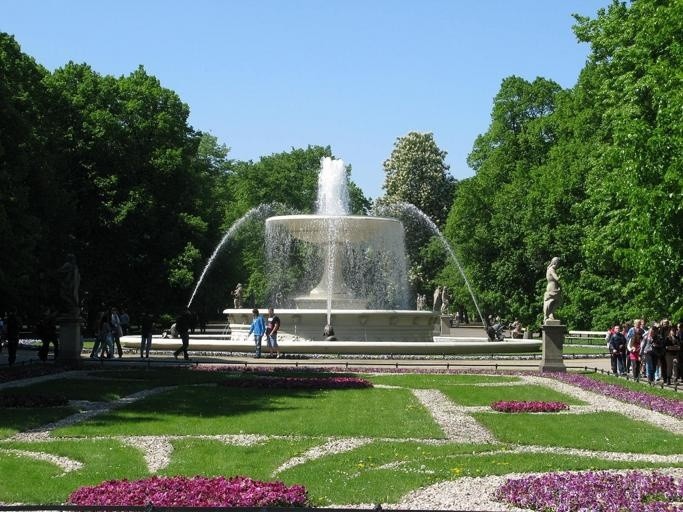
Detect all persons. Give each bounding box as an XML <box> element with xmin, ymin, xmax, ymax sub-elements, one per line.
<box><xmin>433</xmin><ymin>286</ymin><xmax>449</xmax><ymax>315</ymax></box>
<box><xmin>450</xmin><ymin>312</ymin><xmax>523</xmax><ymax>342</ymax></box>
<box><xmin>605</xmin><ymin>319</ymin><xmax>683</xmax><ymax>391</ymax></box>
<box><xmin>544</xmin><ymin>257</ymin><xmax>562</xmax><ymax>320</ymax></box>
<box><xmin>232</xmin><ymin>283</ymin><xmax>243</xmax><ymax>309</ymax></box>
<box><xmin>266</xmin><ymin>308</ymin><xmax>280</xmax><ymax>359</ymax></box>
<box><xmin>0</xmin><ymin>299</ymin><xmax>209</xmax><ymax>364</ymax></box>
<box><xmin>247</xmin><ymin>309</ymin><xmax>265</xmax><ymax>358</ymax></box>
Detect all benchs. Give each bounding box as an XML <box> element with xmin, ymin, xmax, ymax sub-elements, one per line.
<box><xmin>565</xmin><ymin>331</ymin><xmax>608</xmax><ymax>344</ymax></box>
